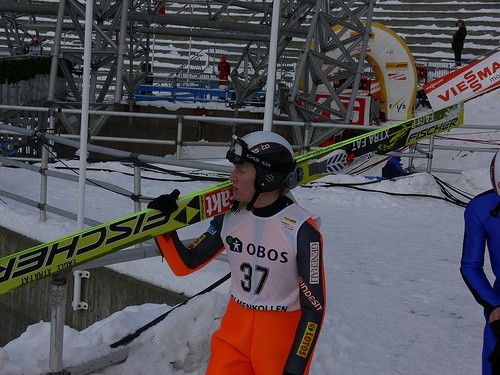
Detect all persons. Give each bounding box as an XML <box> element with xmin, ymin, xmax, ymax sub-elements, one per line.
<box><xmin>382</xmin><ymin>150</ymin><xmax>408</xmax><ymax>177</ymax></box>
<box><xmin>148</xmin><ymin>131</ymin><xmax>327</xmax><ymax>375</ymax></box>
<box><xmin>450</xmin><ymin>20</ymin><xmax>467</xmax><ymax>67</ymax></box>
<box><xmin>460</xmin><ymin>148</ymin><xmax>500</xmax><ymax>375</ymax></box>
<box><xmin>218</xmin><ymin>55</ymin><xmax>230</xmax><ymax>86</ymax></box>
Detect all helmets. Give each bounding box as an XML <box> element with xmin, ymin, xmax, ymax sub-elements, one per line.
<box><xmin>226</xmin><ymin>131</ymin><xmax>295</xmax><ymax>192</ymax></box>
<box><xmin>220</xmin><ymin>56</ymin><xmax>227</xmax><ymax>63</ymax></box>
<box><xmin>490</xmin><ymin>150</ymin><xmax>500</xmax><ymax>196</ymax></box>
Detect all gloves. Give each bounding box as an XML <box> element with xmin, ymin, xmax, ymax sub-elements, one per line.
<box><xmin>147</xmin><ymin>188</ymin><xmax>180</xmax><ymax>215</ymax></box>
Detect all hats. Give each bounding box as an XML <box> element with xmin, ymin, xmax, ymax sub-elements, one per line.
<box><xmin>455</xmin><ymin>19</ymin><xmax>464</xmax><ymax>27</ymax></box>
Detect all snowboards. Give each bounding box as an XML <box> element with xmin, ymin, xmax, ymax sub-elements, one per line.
<box><xmin>0</xmin><ymin>99</ymin><xmax>465</xmax><ymax>297</ymax></box>
